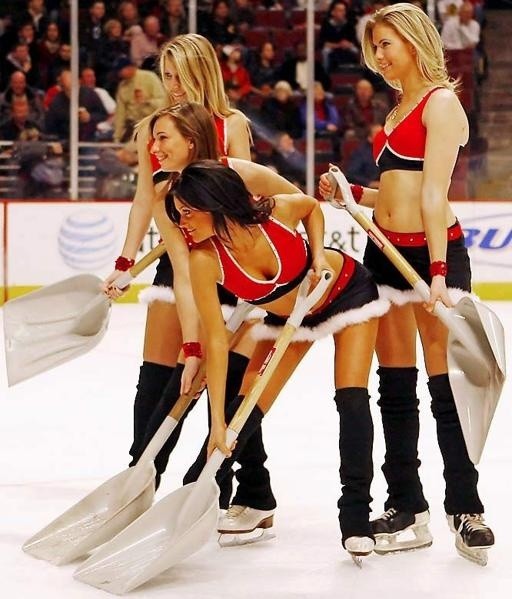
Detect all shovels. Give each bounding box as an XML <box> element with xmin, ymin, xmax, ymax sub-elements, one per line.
<box><xmin>325</xmin><ymin>166</ymin><xmax>506</xmax><ymax>388</ymax></box>
<box><xmin>74</xmin><ymin>268</ymin><xmax>333</xmax><ymax>596</ymax></box>
<box><xmin>22</xmin><ymin>301</ymin><xmax>256</xmax><ymax>567</ymax></box>
<box><xmin>3</xmin><ymin>239</ymin><xmax>167</xmax><ymax>387</ymax></box>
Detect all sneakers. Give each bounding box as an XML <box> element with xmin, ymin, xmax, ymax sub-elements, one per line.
<box><xmin>218</xmin><ymin>505</ymin><xmax>276</xmax><ymax>533</ymax></box>
<box><xmin>341</xmin><ymin>537</ymin><xmax>375</xmax><ymax>555</ymax></box>
<box><xmin>369</xmin><ymin>507</ymin><xmax>431</xmax><ymax>535</ymax></box>
<box><xmin>446</xmin><ymin>511</ymin><xmax>494</xmax><ymax>545</ymax></box>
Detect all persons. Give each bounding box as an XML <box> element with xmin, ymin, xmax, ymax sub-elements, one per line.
<box><xmin>1</xmin><ymin>0</ymin><xmax>488</xmax><ymax>200</ymax></box>
<box><xmin>319</xmin><ymin>1</ymin><xmax>494</xmax><ymax>568</ymax></box>
<box><xmin>103</xmin><ymin>33</ymin><xmax>388</xmax><ymax>569</ymax></box>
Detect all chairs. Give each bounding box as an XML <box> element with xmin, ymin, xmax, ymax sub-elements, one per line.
<box><xmin>246</xmin><ymin>8</ymin><xmax>481</xmax><ymax>198</ymax></box>
